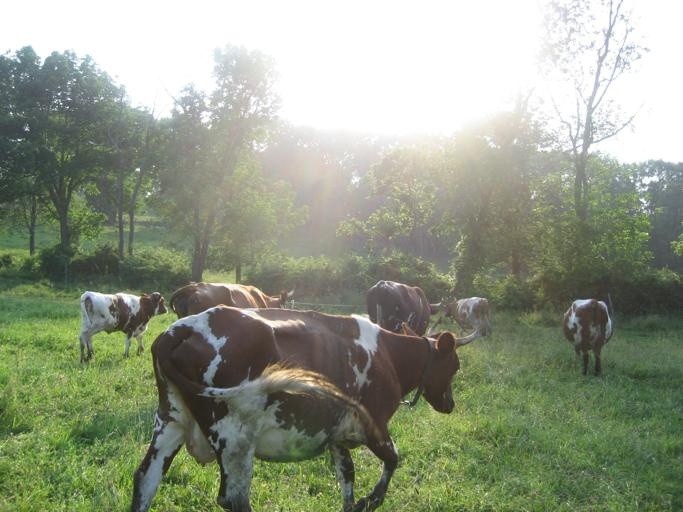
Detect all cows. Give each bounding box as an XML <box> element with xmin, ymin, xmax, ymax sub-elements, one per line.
<box><xmin>562</xmin><ymin>297</ymin><xmax>613</xmax><ymax>377</ymax></box>
<box><xmin>130</xmin><ymin>302</ymin><xmax>481</xmax><ymax>512</ymax></box>
<box><xmin>169</xmin><ymin>280</ymin><xmax>294</xmax><ymax>320</ymax></box>
<box><xmin>441</xmin><ymin>295</ymin><xmax>494</xmax><ymax>339</ymax></box>
<box><xmin>79</xmin><ymin>290</ymin><xmax>168</xmax><ymax>363</ymax></box>
<box><xmin>365</xmin><ymin>279</ymin><xmax>444</xmax><ymax>338</ymax></box>
